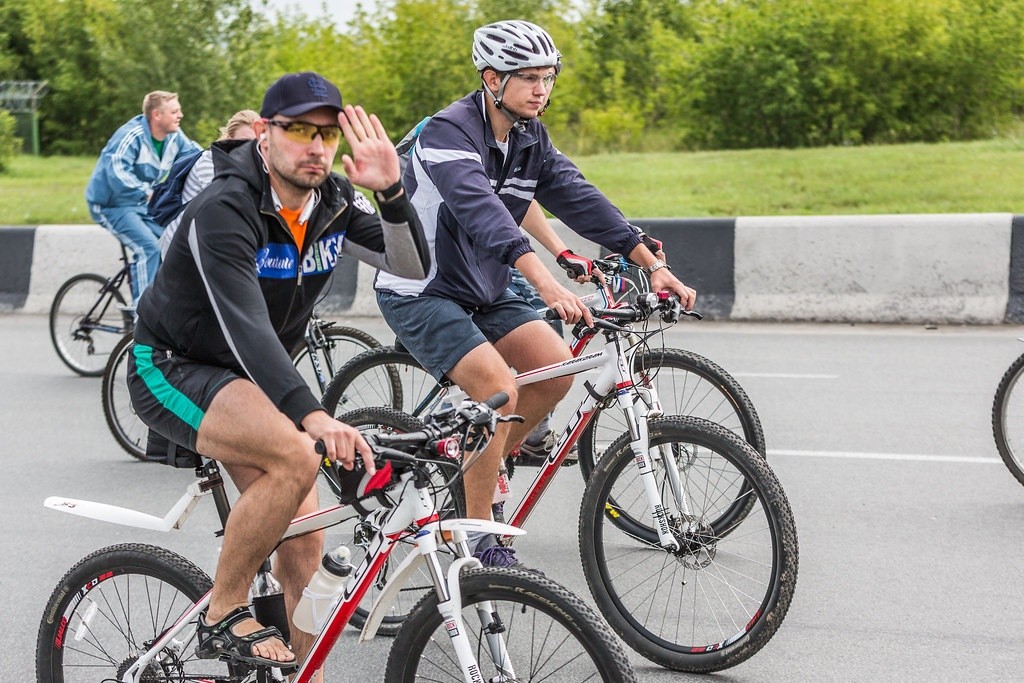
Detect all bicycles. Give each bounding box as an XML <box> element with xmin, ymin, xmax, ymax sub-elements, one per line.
<box><xmin>32</xmin><ymin>392</ymin><xmax>641</xmax><ymax>683</ymax></box>
<box><xmin>101</xmin><ymin>266</ymin><xmax>404</xmax><ymax>467</ymax></box>
<box><xmin>46</xmin><ymin>240</ymin><xmax>137</xmax><ymax>379</ymax></box>
<box><xmin>313</xmin><ymin>254</ymin><xmax>802</xmax><ymax>673</ymax></box>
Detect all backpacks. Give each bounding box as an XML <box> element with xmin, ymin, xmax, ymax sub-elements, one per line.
<box><xmin>147</xmin><ymin>147</ymin><xmax>220</xmax><ymax>228</ymax></box>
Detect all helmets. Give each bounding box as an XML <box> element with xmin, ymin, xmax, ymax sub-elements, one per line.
<box><xmin>472</xmin><ymin>20</ymin><xmax>563</xmax><ymax>72</ymax></box>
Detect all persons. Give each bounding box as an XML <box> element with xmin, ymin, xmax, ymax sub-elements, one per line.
<box><xmin>373</xmin><ymin>18</ymin><xmax>699</xmax><ymax>579</ymax></box>
<box><xmin>124</xmin><ymin>71</ymin><xmax>432</xmax><ymax>683</ymax></box>
<box><xmin>83</xmin><ymin>90</ymin><xmax>195</xmax><ymax>320</ymax></box>
<box><xmin>157</xmin><ymin>108</ymin><xmax>262</xmax><ymax>278</ymax></box>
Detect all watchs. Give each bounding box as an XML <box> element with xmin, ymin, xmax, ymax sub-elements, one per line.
<box><xmin>648</xmin><ymin>258</ymin><xmax>672</xmax><ymax>274</ymax></box>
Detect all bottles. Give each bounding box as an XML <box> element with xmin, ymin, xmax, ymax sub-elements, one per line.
<box><xmin>251</xmin><ymin>557</ymin><xmax>292</xmax><ymax>650</ymax></box>
<box><xmin>291</xmin><ymin>545</ymin><xmax>352</xmax><ymax>637</ymax></box>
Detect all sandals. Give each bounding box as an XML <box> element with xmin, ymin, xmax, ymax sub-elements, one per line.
<box><xmin>194</xmin><ymin>610</ymin><xmax>298</xmax><ymax>669</ymax></box>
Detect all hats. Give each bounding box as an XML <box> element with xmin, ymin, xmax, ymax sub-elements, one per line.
<box><xmin>260</xmin><ymin>74</ymin><xmax>346</xmax><ymax>118</ymax></box>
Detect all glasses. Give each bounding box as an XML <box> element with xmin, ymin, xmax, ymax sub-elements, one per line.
<box><xmin>268</xmin><ymin>118</ymin><xmax>344</xmax><ymax>146</ymax></box>
<box><xmin>504</xmin><ymin>72</ymin><xmax>558</xmax><ymax>89</ymax></box>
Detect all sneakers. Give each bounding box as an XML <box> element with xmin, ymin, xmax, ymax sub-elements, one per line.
<box><xmin>518</xmin><ymin>430</ymin><xmax>579</xmax><ymax>460</ymax></box>
<box><xmin>467</xmin><ymin>531</ymin><xmax>545</xmax><ymax>578</ymax></box>
